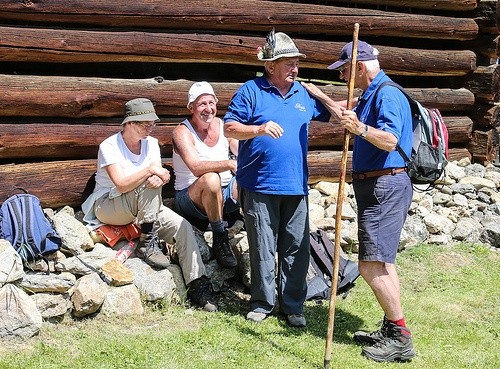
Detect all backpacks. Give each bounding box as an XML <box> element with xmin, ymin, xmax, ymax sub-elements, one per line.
<box><xmin>374</xmin><ymin>81</ymin><xmax>448</xmax><ymax>184</ymax></box>
<box><xmin>0</xmin><ymin>187</ymin><xmax>61</xmax><ymax>271</ymax></box>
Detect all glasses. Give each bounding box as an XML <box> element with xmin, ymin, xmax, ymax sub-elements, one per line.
<box><xmin>138</xmin><ymin>121</ymin><xmax>156</xmax><ymax>129</ymax></box>
<box><xmin>339</xmin><ymin>63</ymin><xmax>351</xmax><ymax>73</ymax></box>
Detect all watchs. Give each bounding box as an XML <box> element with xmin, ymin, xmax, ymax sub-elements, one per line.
<box><xmin>359</xmin><ymin>124</ymin><xmax>368</xmax><ymax>138</ymax></box>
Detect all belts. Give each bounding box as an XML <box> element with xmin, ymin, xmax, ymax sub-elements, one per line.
<box><xmin>351</xmin><ymin>166</ymin><xmax>406</xmax><ymax>181</ymax></box>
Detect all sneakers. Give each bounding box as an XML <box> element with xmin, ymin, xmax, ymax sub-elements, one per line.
<box><xmin>354</xmin><ymin>314</ymin><xmax>397</xmax><ymax>345</ymax></box>
<box><xmin>288</xmin><ymin>314</ymin><xmax>307</xmax><ymax>326</ymax></box>
<box><xmin>362</xmin><ymin>322</ymin><xmax>415</xmax><ymax>362</ymax></box>
<box><xmin>246</xmin><ymin>310</ymin><xmax>267</xmax><ymax>321</ymax></box>
<box><xmin>189</xmin><ymin>288</ymin><xmax>216</xmax><ymax>311</ymax></box>
<box><xmin>213</xmin><ymin>230</ymin><xmax>238</xmax><ymax>269</ymax></box>
<box><xmin>136</xmin><ymin>233</ymin><xmax>171</xmax><ymax>269</ymax></box>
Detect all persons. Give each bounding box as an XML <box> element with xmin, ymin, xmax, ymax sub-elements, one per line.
<box><xmin>81</xmin><ymin>97</ymin><xmax>212</xmax><ymax>305</ymax></box>
<box><xmin>225</xmin><ymin>33</ymin><xmax>361</xmax><ymax>325</ymax></box>
<box><xmin>170</xmin><ymin>81</ymin><xmax>241</xmax><ymax>268</ymax></box>
<box><xmin>299</xmin><ymin>41</ymin><xmax>414</xmax><ymax>363</ymax></box>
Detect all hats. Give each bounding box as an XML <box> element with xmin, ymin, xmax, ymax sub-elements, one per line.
<box><xmin>257</xmin><ymin>27</ymin><xmax>307</xmax><ymax>61</ymax></box>
<box><xmin>187</xmin><ymin>82</ymin><xmax>219</xmax><ymax>104</ymax></box>
<box><xmin>327</xmin><ymin>39</ymin><xmax>378</xmax><ymax>70</ymax></box>
<box><xmin>121</xmin><ymin>97</ymin><xmax>161</xmax><ymax>125</ymax></box>
